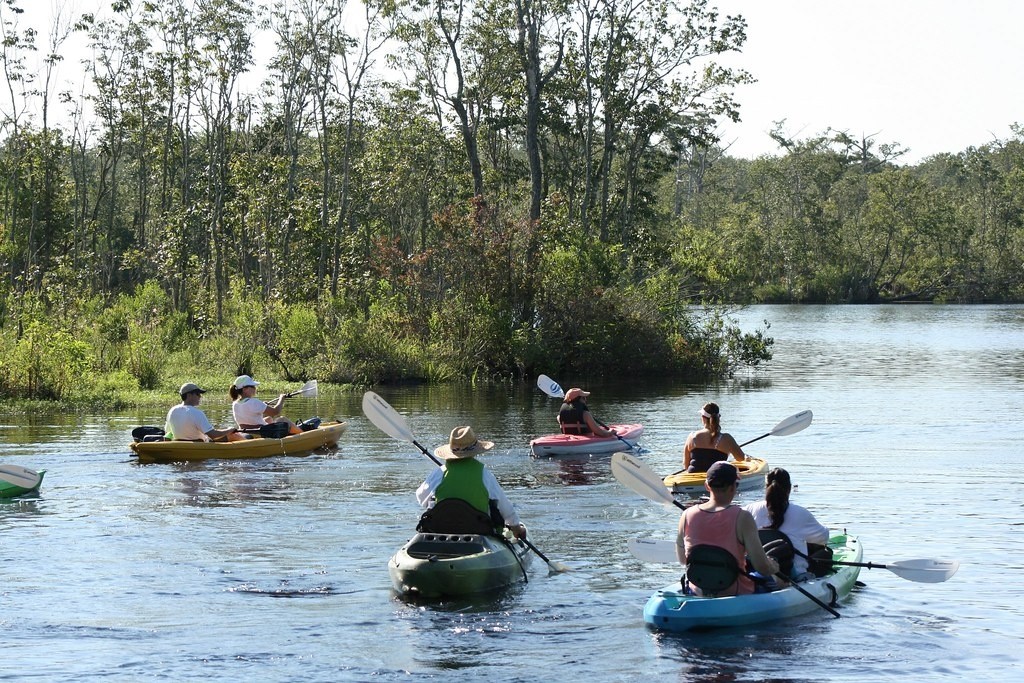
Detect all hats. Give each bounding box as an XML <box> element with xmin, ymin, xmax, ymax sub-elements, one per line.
<box><xmin>179</xmin><ymin>381</ymin><xmax>207</xmax><ymax>395</ymax></box>
<box><xmin>564</xmin><ymin>387</ymin><xmax>590</xmax><ymax>402</ymax></box>
<box><xmin>233</xmin><ymin>374</ymin><xmax>261</xmax><ymax>389</ymax></box>
<box><xmin>434</xmin><ymin>425</ymin><xmax>494</xmax><ymax>460</ymax></box>
<box><xmin>698</xmin><ymin>406</ymin><xmax>721</xmax><ymax>419</ymax></box>
<box><xmin>706</xmin><ymin>460</ymin><xmax>741</xmax><ymax>488</ymax></box>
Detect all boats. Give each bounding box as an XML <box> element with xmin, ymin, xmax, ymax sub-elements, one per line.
<box><xmin>529</xmin><ymin>423</ymin><xmax>645</xmax><ymax>458</ymax></box>
<box><xmin>641</xmin><ymin>527</ymin><xmax>864</xmax><ymax>632</ymax></box>
<box><xmin>387</xmin><ymin>519</ymin><xmax>536</xmax><ymax>599</ymax></box>
<box><xmin>0</xmin><ymin>468</ymin><xmax>45</xmax><ymax>499</ymax></box>
<box><xmin>662</xmin><ymin>454</ymin><xmax>769</xmax><ymax>493</ymax></box>
<box><xmin>129</xmin><ymin>420</ymin><xmax>348</xmax><ymax>461</ymax></box>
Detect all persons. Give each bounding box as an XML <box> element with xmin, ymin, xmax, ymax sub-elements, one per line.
<box><xmin>229</xmin><ymin>375</ymin><xmax>303</xmax><ymax>440</ymax></box>
<box><xmin>556</xmin><ymin>387</ymin><xmax>617</xmax><ymax>439</ymax></box>
<box><xmin>416</xmin><ymin>425</ymin><xmax>527</xmax><ymax>542</ymax></box>
<box><xmin>164</xmin><ymin>383</ymin><xmax>248</xmax><ymax>442</ymax></box>
<box><xmin>683</xmin><ymin>403</ymin><xmax>745</xmax><ymax>474</ymax></box>
<box><xmin>674</xmin><ymin>461</ymin><xmax>790</xmax><ymax>595</ymax></box>
<box><xmin>740</xmin><ymin>467</ymin><xmax>829</xmax><ymax>590</ymax></box>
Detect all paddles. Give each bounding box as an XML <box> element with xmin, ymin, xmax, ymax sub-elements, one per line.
<box><xmin>267</xmin><ymin>378</ymin><xmax>319</xmax><ymax>403</ymax></box>
<box><xmin>626</xmin><ymin>537</ymin><xmax>961</xmax><ymax>585</ymax></box>
<box><xmin>661</xmin><ymin>409</ymin><xmax>813</xmax><ymax>481</ymax></box>
<box><xmin>537</xmin><ymin>373</ymin><xmax>647</xmax><ymax>453</ymax></box>
<box><xmin>131</xmin><ymin>421</ymin><xmax>290</xmax><ymax>443</ymax></box>
<box><xmin>611</xmin><ymin>451</ymin><xmax>844</xmax><ymax>618</ymax></box>
<box><xmin>360</xmin><ymin>390</ymin><xmax>564</xmax><ymax>572</ymax></box>
